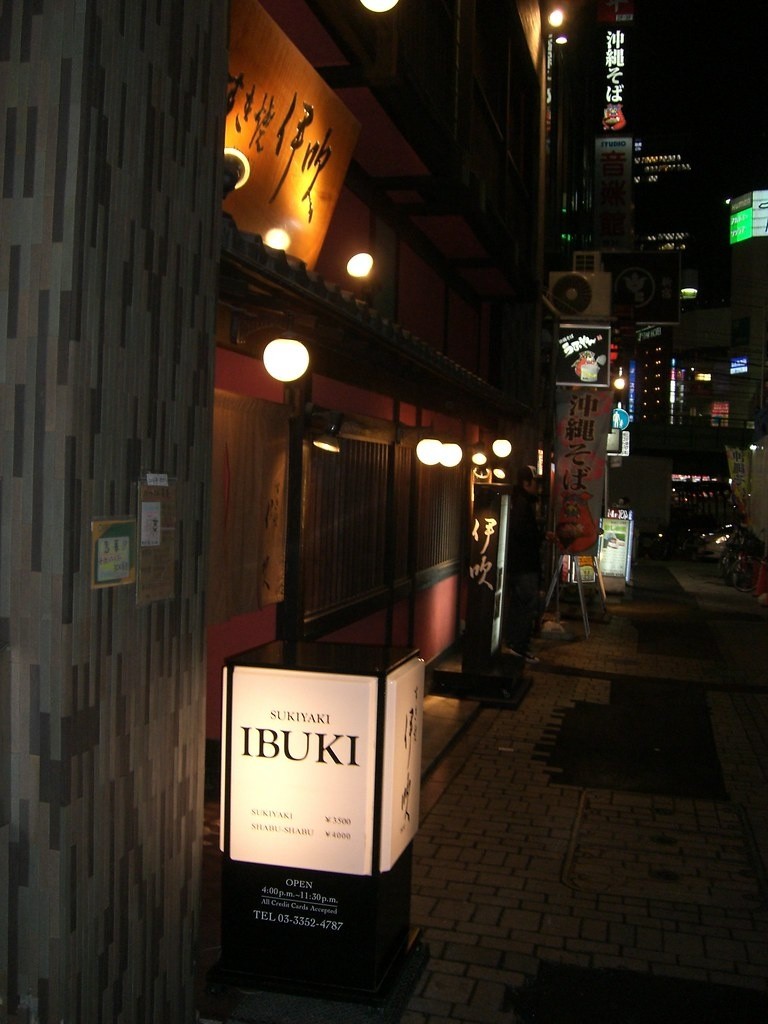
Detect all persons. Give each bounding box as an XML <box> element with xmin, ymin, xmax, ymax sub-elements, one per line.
<box><xmin>506</xmin><ymin>464</ymin><xmax>558</xmax><ymax>665</ymax></box>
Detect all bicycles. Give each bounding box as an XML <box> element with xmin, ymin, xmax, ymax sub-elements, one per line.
<box><xmin>722</xmin><ymin>532</ymin><xmax>761</xmax><ymax>591</ymax></box>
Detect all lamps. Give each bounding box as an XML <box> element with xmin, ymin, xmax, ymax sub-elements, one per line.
<box><xmin>306</xmin><ymin>401</ymin><xmax>345</xmax><ymax>454</ymax></box>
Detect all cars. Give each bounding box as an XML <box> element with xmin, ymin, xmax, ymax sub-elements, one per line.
<box><xmin>697</xmin><ymin>524</ymin><xmax>733</xmax><ymax>560</ymax></box>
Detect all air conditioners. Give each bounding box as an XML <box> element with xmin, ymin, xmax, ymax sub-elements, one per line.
<box><xmin>572</xmin><ymin>249</ymin><xmax>601</xmax><ymax>273</ymax></box>
<box><xmin>548</xmin><ymin>270</ymin><xmax>613</xmax><ymax>319</ymax></box>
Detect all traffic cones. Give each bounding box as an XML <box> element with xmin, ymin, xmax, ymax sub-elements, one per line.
<box><xmin>753</xmin><ymin>564</ymin><xmax>768</xmax><ymax>597</ymax></box>
<box><xmin>736</xmin><ymin>553</ymin><xmax>746</xmax><ymax>573</ymax></box>
<box><xmin>752</xmin><ymin>559</ymin><xmax>758</xmax><ymax>585</ymax></box>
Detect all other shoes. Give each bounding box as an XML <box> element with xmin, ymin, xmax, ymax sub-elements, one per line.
<box><xmin>506</xmin><ymin>648</ymin><xmax>540</xmax><ymax>664</ymax></box>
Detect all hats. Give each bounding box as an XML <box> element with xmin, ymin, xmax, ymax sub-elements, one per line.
<box><xmin>517</xmin><ymin>464</ymin><xmax>544</xmax><ymax>481</ymax></box>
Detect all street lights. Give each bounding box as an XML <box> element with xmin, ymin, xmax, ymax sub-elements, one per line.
<box><xmin>491</xmin><ymin>430</ymin><xmax>514</xmax><ymax>480</ymax></box>
<box><xmin>262</xmin><ymin>337</ymin><xmax>345</xmax><ymax>640</ymax></box>
